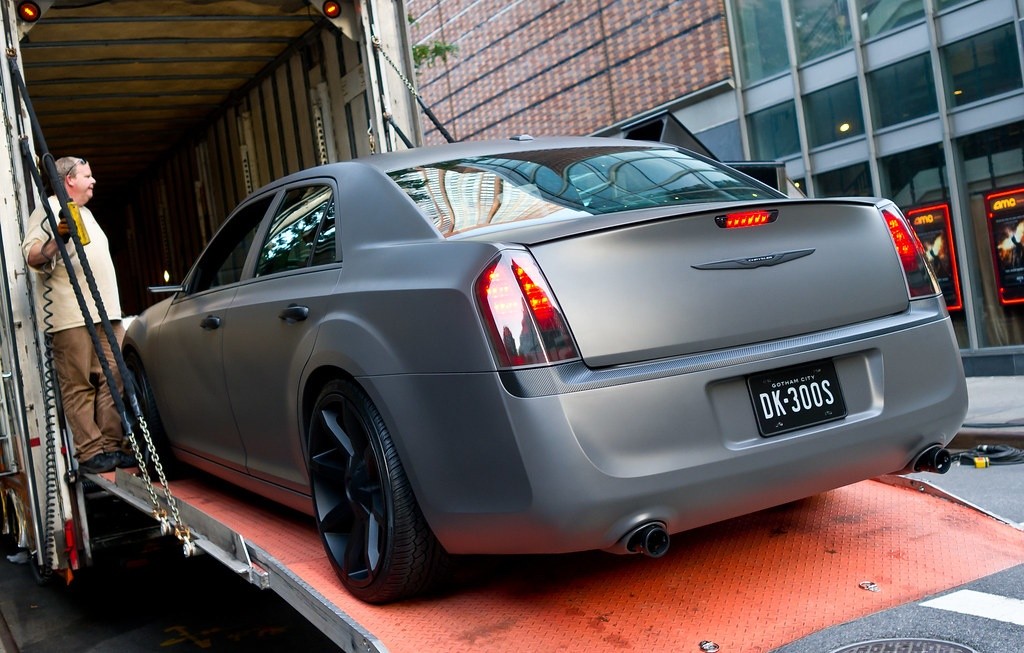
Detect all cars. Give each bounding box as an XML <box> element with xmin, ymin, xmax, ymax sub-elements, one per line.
<box><xmin>119</xmin><ymin>134</ymin><xmax>971</xmax><ymax>608</ymax></box>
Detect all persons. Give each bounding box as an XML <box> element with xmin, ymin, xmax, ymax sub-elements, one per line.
<box><xmin>23</xmin><ymin>157</ymin><xmax>139</xmax><ymax>475</ymax></box>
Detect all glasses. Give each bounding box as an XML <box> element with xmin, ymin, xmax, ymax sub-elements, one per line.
<box><xmin>66</xmin><ymin>158</ymin><xmax>87</xmax><ymax>175</ymax></box>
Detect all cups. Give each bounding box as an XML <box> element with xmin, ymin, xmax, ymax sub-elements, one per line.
<box><xmin>66</xmin><ymin>201</ymin><xmax>91</xmax><ymax>246</ymax></box>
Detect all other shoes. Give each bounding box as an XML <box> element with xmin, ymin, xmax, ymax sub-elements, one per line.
<box><xmin>106</xmin><ymin>450</ymin><xmax>145</xmax><ymax>467</ymax></box>
<box><xmin>78</xmin><ymin>452</ymin><xmax>121</xmax><ymax>473</ymax></box>
